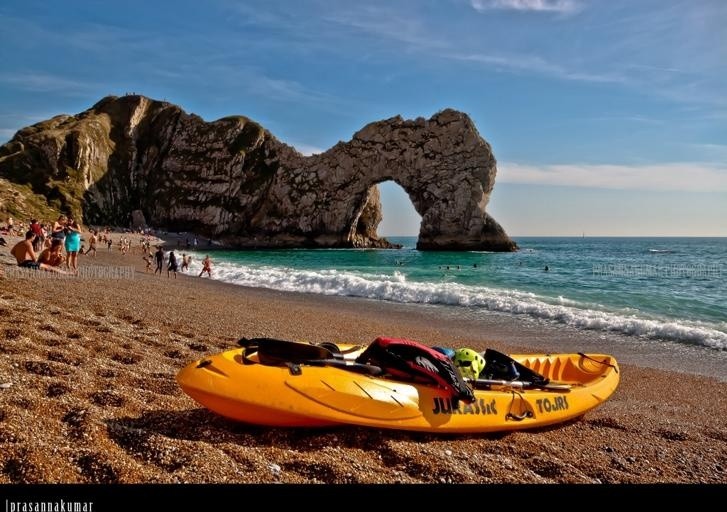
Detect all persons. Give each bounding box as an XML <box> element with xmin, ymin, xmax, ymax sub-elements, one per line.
<box><xmin>6</xmin><ymin>214</ymin><xmax>214</xmax><ymax>279</ymax></box>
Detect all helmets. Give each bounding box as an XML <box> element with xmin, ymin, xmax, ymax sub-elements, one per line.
<box><xmin>451</xmin><ymin>347</ymin><xmax>487</xmax><ymax>380</ymax></box>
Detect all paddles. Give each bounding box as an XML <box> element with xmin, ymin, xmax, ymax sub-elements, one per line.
<box><xmin>258</xmin><ymin>338</ymin><xmax>534</xmax><ymax>388</ymax></box>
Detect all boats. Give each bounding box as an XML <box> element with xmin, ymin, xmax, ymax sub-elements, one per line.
<box><xmin>175</xmin><ymin>335</ymin><xmax>622</xmax><ymax>434</ymax></box>
